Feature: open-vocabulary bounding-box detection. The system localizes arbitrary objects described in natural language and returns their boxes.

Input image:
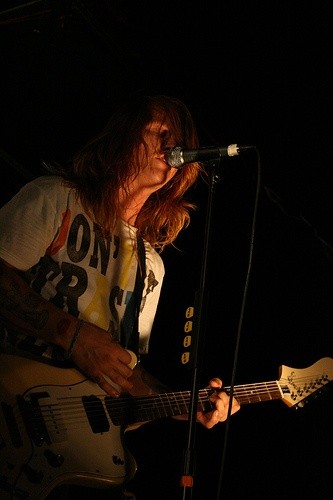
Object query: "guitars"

[1,355,333,496]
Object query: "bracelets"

[63,318,85,363]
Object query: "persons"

[0,93,242,500]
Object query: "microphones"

[164,143,258,169]
